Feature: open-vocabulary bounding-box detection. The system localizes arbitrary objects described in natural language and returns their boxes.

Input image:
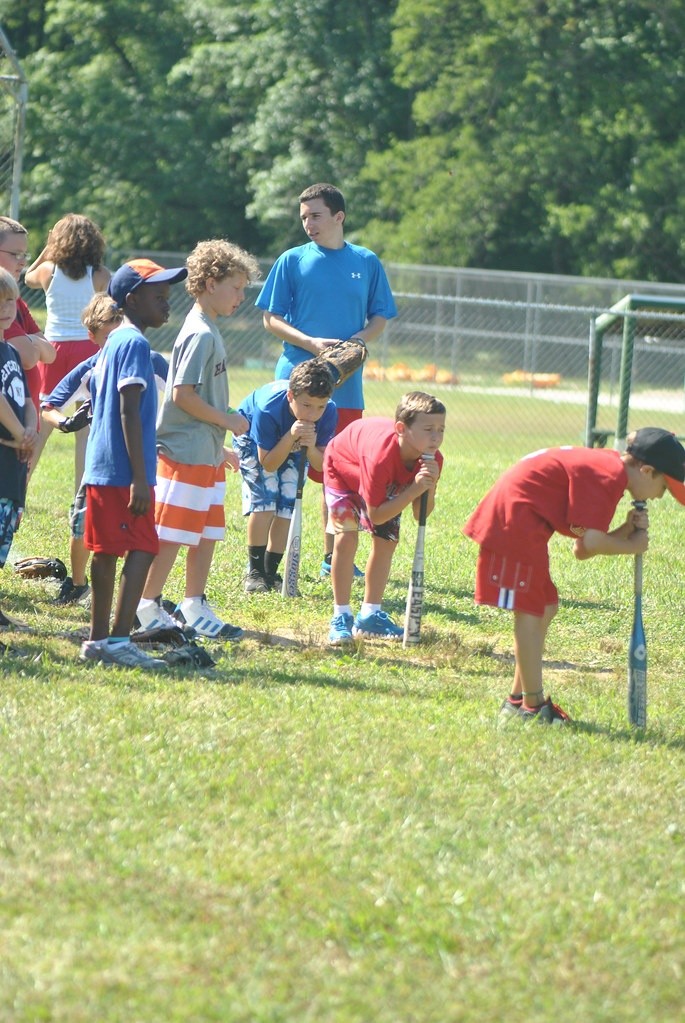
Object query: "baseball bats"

[628,501,647,726]
[403,455,437,644]
[281,446,308,599]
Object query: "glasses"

[0,249,32,262]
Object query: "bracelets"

[227,408,237,414]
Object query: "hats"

[628,426,685,509]
[108,259,189,307]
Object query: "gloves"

[59,398,92,433]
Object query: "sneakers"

[172,593,243,639]
[319,560,331,577]
[79,640,104,662]
[99,640,169,670]
[328,613,355,645]
[516,693,573,727]
[353,564,366,577]
[264,571,283,592]
[52,576,90,607]
[498,694,522,721]
[352,611,409,641]
[237,568,269,592]
[130,596,197,640]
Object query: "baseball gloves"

[319,338,368,387]
[156,641,217,670]
[13,556,67,580]
[131,625,198,648]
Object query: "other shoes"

[0,641,30,659]
[0,610,30,631]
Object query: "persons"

[0,265,38,655]
[39,292,240,610]
[463,428,685,725]
[0,216,57,533]
[322,391,446,646]
[79,258,171,669]
[131,239,263,641]
[231,359,338,591]
[254,183,398,577]
[24,213,111,497]
